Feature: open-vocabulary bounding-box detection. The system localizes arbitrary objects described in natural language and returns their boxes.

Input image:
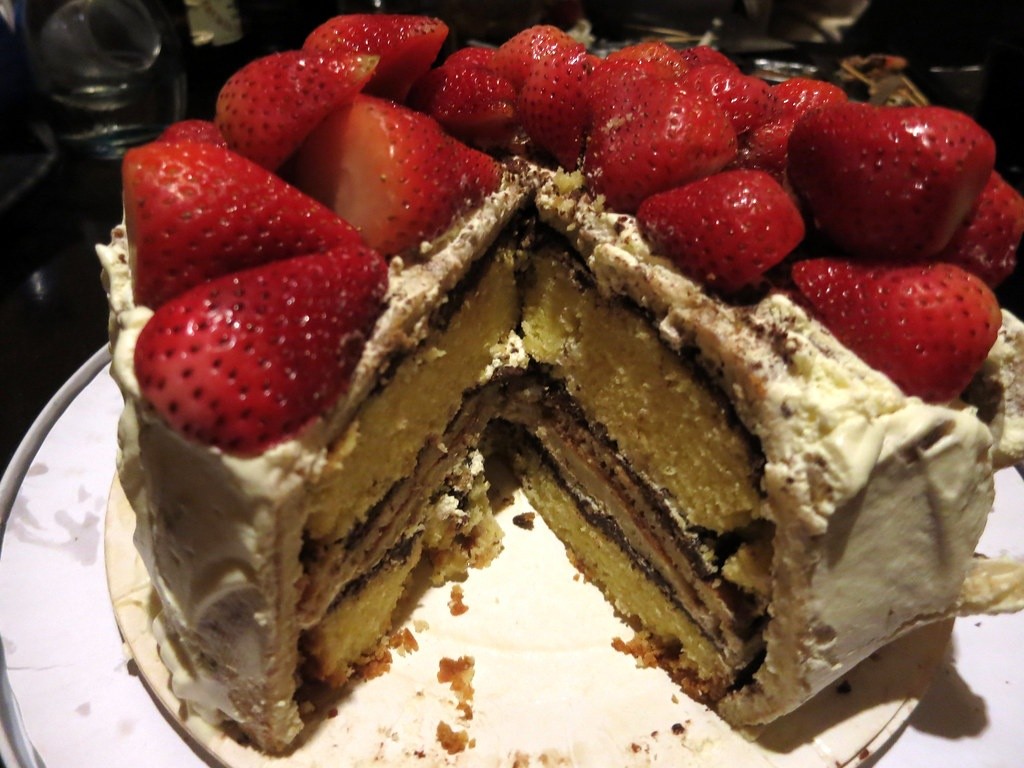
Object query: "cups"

[615,1,732,59]
[31,0,192,161]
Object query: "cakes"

[93,16,1024,752]
